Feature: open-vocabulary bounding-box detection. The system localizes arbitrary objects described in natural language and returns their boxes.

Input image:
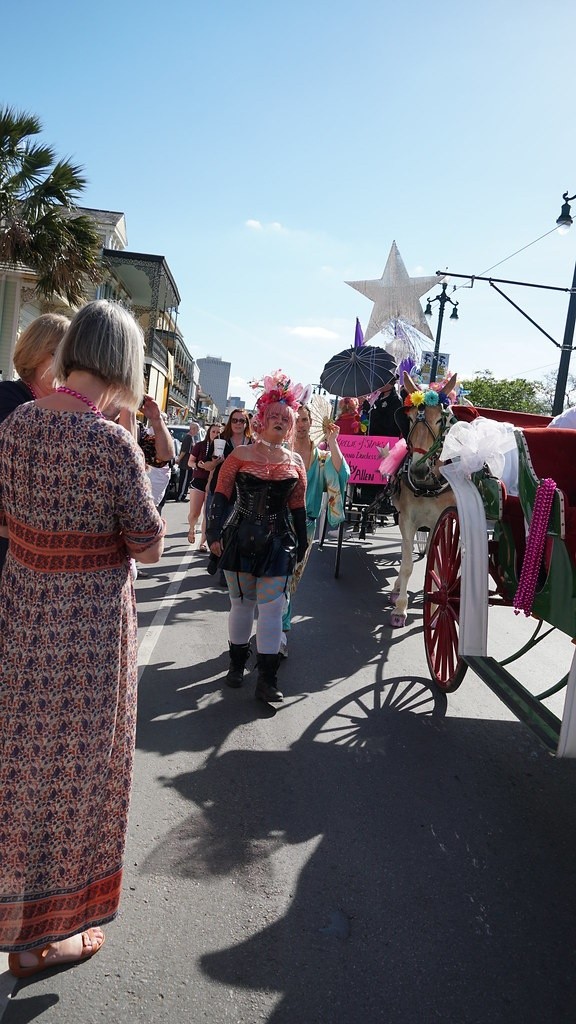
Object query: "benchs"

[448,405,554,521]
[512,428,576,540]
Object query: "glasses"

[232,418,247,424]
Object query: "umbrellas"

[320,346,396,397]
[355,323,363,347]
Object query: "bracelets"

[262,439,281,448]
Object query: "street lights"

[313,383,327,397]
[551,192,576,417]
[422,284,459,384]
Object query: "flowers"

[402,383,458,415]
[246,367,303,435]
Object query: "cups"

[215,438,226,459]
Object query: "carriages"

[316,370,475,577]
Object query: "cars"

[164,425,206,498]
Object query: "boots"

[255,652,285,702]
[226,641,253,688]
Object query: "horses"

[390,370,458,628]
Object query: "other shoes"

[176,498,189,502]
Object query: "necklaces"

[57,387,105,418]
[206,441,210,456]
[26,383,37,400]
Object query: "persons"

[334,374,404,436]
[172,394,350,702]
[0,301,177,976]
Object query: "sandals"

[188,530,195,543]
[200,544,207,552]
[9,928,105,978]
